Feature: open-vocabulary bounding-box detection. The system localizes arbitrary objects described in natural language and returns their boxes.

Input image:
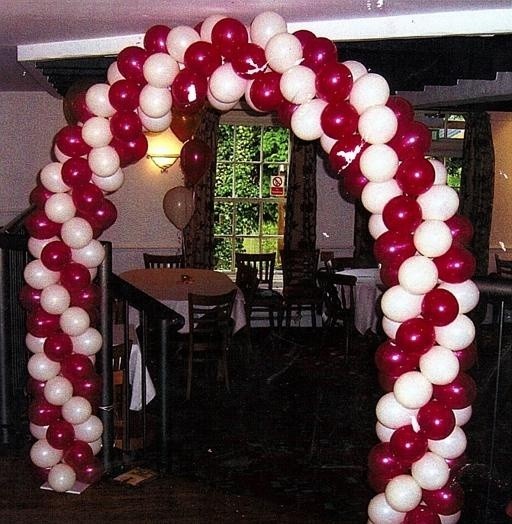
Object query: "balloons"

[366,256,481,524]
[138,9,369,140]
[27,395,104,492]
[32,47,149,233]
[19,217,104,406]
[318,73,474,284]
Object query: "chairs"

[232,249,373,365]
[490,254,512,338]
[186,288,239,399]
[140,252,190,268]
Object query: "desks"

[332,267,384,341]
[118,266,244,393]
